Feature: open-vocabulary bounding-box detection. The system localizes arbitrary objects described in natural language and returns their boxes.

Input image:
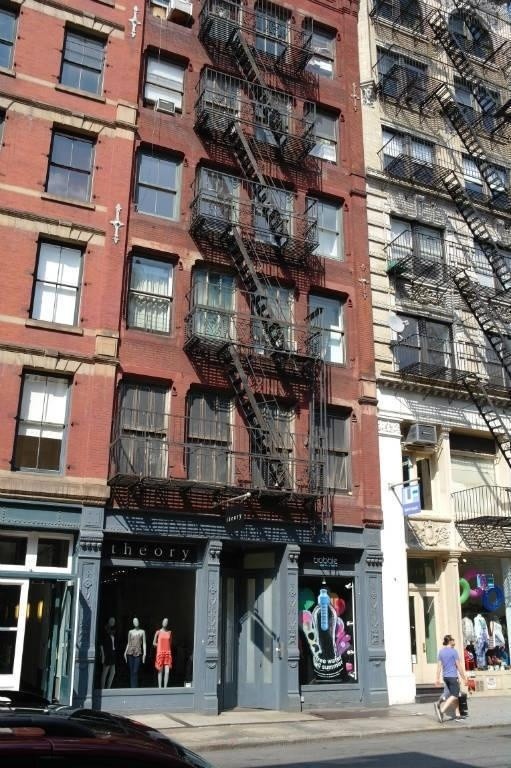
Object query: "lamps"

[313,559,327,584]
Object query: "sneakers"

[455,715,466,722]
[433,701,447,723]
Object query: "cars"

[1,689,219,768]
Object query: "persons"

[434,634,470,723]
[463,616,475,644]
[473,614,490,643]
[490,615,505,647]
[152,617,173,688]
[98,617,118,689]
[123,617,146,687]
